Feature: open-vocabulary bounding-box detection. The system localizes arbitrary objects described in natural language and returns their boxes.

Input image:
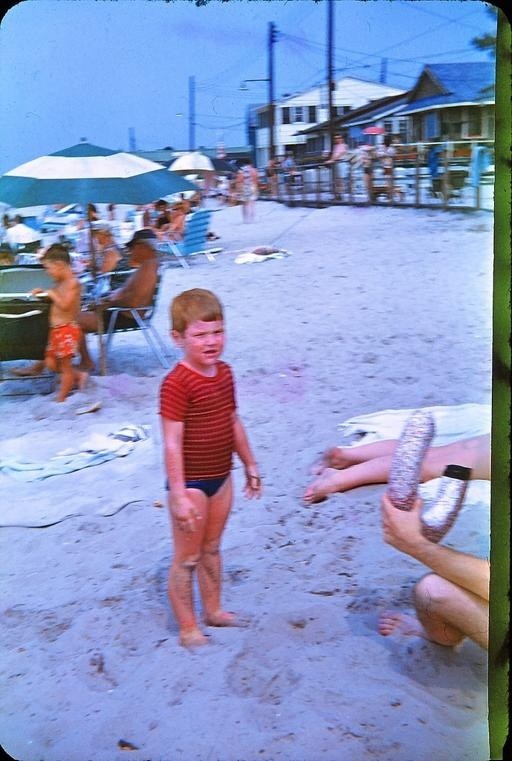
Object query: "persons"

[378,493,490,651]
[160,288,262,652]
[261,153,298,172]
[0,192,218,290]
[303,432,491,506]
[10,229,159,376]
[235,158,260,222]
[327,133,445,202]
[32,243,89,402]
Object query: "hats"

[126,229,157,247]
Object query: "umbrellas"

[0,136,203,276]
[169,146,239,177]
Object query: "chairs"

[160,206,218,267]
[104,259,174,368]
[0,291,54,395]
